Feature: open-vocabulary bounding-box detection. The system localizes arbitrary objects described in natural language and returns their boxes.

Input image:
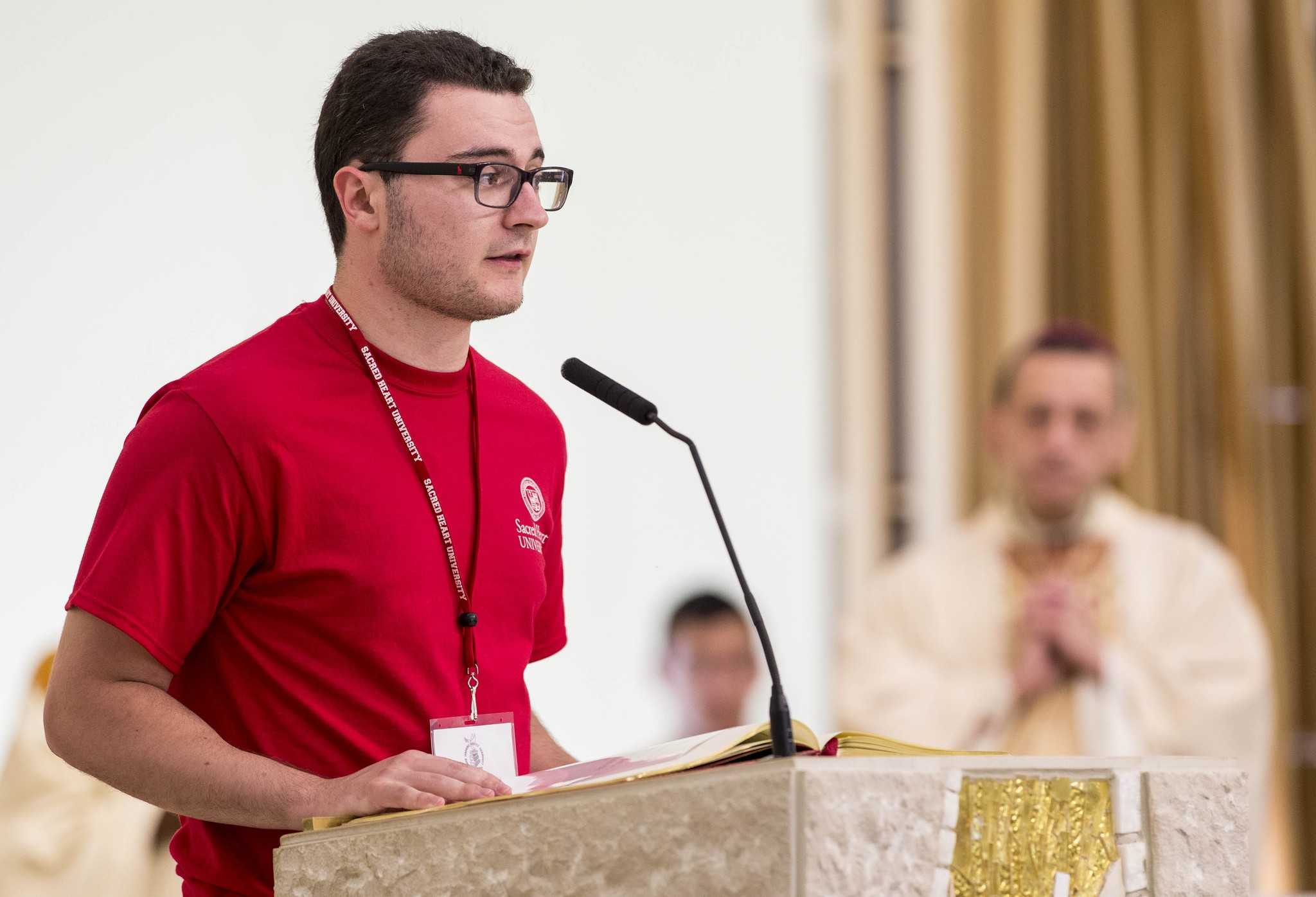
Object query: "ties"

[1006,536,1108,757]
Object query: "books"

[345,714,1012,824]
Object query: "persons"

[661,594,757,737]
[47,26,579,897]
[833,325,1279,768]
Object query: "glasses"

[354,161,574,212]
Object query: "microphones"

[559,356,795,761]
